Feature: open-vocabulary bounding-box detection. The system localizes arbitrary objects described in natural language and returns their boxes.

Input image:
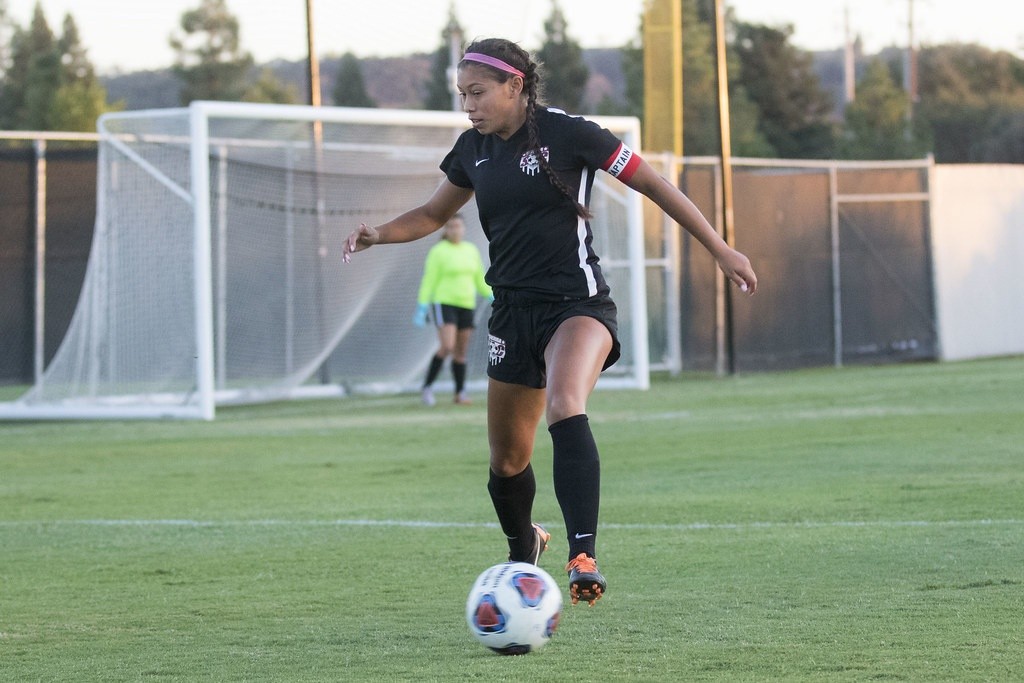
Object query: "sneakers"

[508,522,550,566]
[564,553,606,607]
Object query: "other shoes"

[423,385,437,405]
[455,395,472,405]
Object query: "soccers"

[462,560,565,657]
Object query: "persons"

[340,40,757,605]
[413,213,495,407]
